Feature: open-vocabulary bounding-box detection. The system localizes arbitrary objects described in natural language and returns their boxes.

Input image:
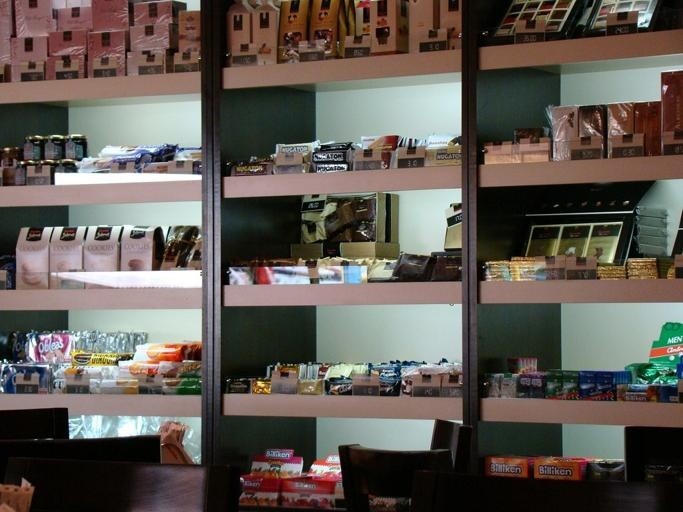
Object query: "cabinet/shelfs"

[0,0,202,466]
[476,0,682,464]
[221,0,463,471]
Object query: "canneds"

[3,134,88,186]
